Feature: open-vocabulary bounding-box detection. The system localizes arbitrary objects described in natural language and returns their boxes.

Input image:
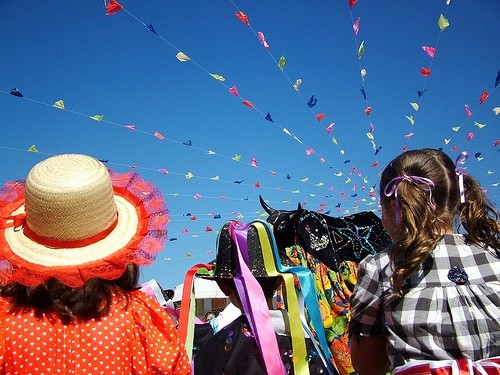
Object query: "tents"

[171,277,231,301]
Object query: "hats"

[0,153,168,287]
[195,221,302,278]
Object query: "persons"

[346,149,500,375]
[193,219,331,375]
[0,152,194,374]
[162,288,284,338]
